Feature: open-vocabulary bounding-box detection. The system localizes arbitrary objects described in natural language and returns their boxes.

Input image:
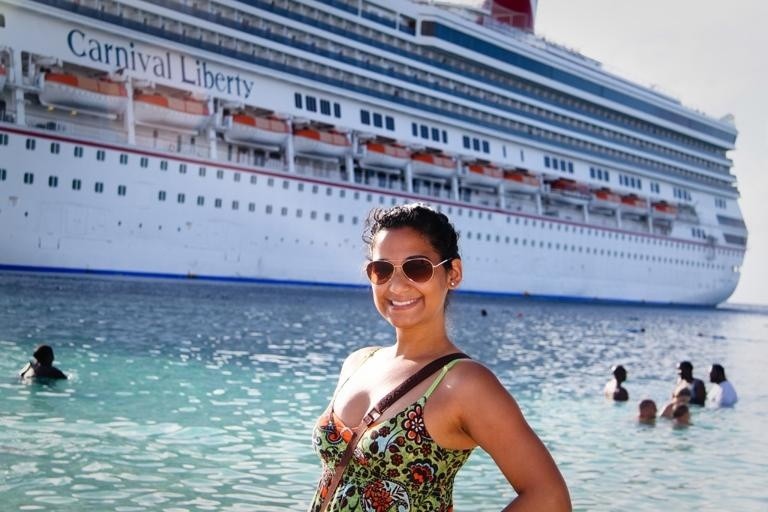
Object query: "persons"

[604,362,737,430]
[308,202,572,512]
[18,345,67,383]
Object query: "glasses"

[364,257,452,286]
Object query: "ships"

[1,0,750,311]
